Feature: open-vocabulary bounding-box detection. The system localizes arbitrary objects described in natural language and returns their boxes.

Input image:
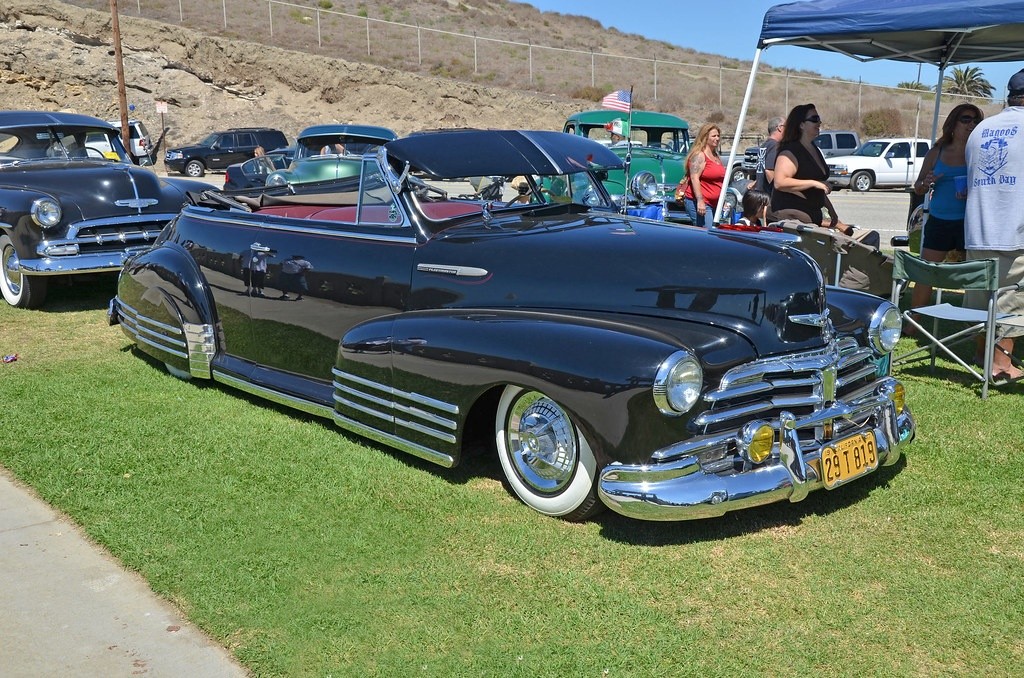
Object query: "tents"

[713,0,1024,228]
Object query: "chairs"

[420,202,484,219]
[767,221,893,304]
[304,205,400,224]
[884,244,1024,397]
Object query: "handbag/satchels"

[676,179,689,207]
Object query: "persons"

[320,144,350,155]
[734,189,770,226]
[964,68,1024,379]
[254,146,276,174]
[683,124,726,228]
[277,254,314,301]
[470,175,503,201]
[606,117,628,137]
[602,89,631,113]
[611,132,630,145]
[238,244,277,297]
[900,104,985,336]
[747,103,854,236]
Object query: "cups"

[954,176,967,195]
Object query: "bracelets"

[921,180,925,188]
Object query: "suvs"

[163,127,290,177]
[84,120,153,158]
[744,131,861,192]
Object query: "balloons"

[129,104,135,111]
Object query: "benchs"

[250,205,337,220]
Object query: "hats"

[604,118,627,136]
[511,176,529,190]
[1008,69,1024,90]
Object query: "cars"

[529,108,692,224]
[0,110,223,310]
[223,124,398,191]
[824,138,932,193]
[107,128,916,524]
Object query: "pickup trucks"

[667,139,748,187]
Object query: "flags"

[610,228,636,236]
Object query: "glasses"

[804,115,820,123]
[957,115,982,124]
